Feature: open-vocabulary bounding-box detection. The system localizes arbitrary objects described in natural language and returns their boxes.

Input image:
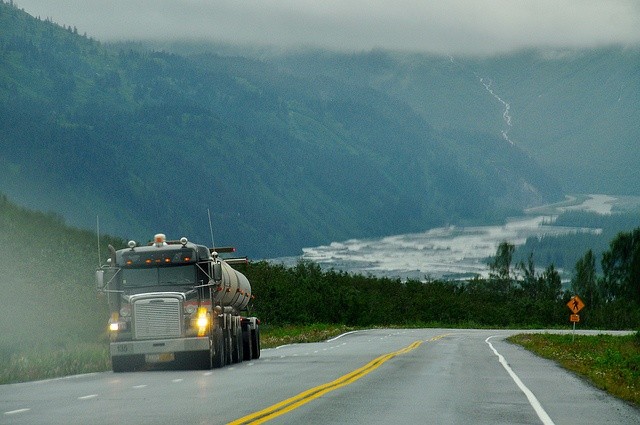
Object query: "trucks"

[95,207,260,372]
